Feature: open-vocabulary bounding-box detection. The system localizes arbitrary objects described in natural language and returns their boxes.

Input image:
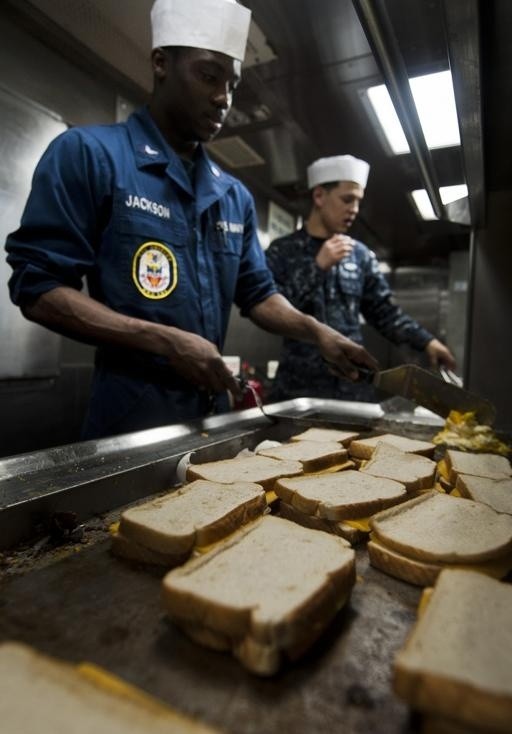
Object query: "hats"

[150,0,252,63]
[307,154,371,190]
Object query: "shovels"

[234,378,369,433]
[279,342,496,427]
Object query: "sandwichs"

[111,426,512,734]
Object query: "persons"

[259,151,461,409]
[2,0,381,447]
